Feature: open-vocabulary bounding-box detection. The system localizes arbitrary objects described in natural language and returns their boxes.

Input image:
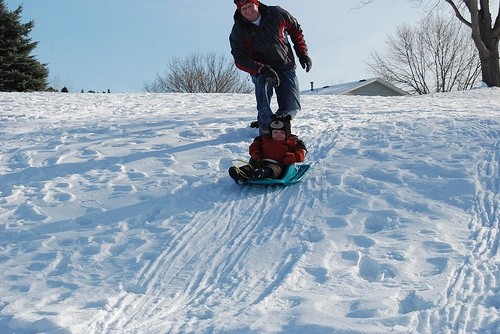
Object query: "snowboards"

[246,164,312,187]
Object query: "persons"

[229,0,313,134]
[229,115,308,181]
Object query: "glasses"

[241,4,256,10]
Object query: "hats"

[234,0,259,11]
[268,114,292,140]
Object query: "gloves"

[260,65,280,87]
[282,152,296,165]
[299,55,312,72]
[252,152,260,160]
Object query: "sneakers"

[228,166,246,185]
[252,168,265,180]
